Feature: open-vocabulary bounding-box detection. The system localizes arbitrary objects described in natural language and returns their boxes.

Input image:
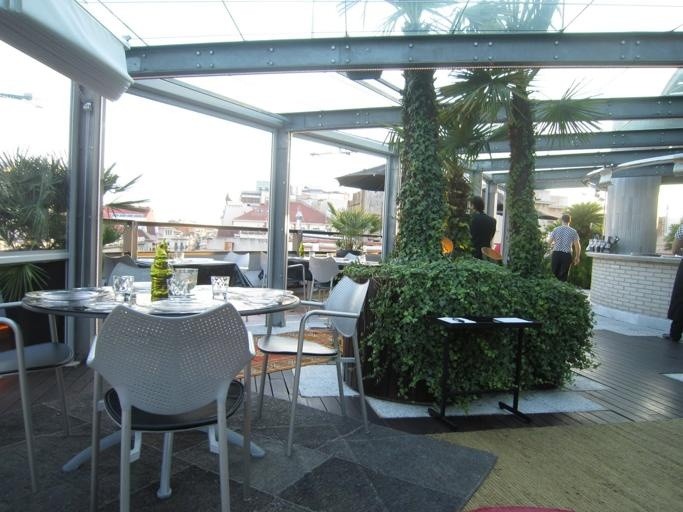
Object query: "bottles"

[588,235,612,253]
[299,241,304,257]
[150,240,172,301]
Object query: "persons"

[467,196,497,261]
[659,218,683,342]
[546,214,581,283]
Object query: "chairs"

[480,245,503,267]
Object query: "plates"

[25,288,108,304]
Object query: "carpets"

[0,384,497,512]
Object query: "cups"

[210,275,231,302]
[110,274,135,303]
[174,266,198,298]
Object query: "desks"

[426,313,542,426]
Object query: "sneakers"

[663,334,678,344]
